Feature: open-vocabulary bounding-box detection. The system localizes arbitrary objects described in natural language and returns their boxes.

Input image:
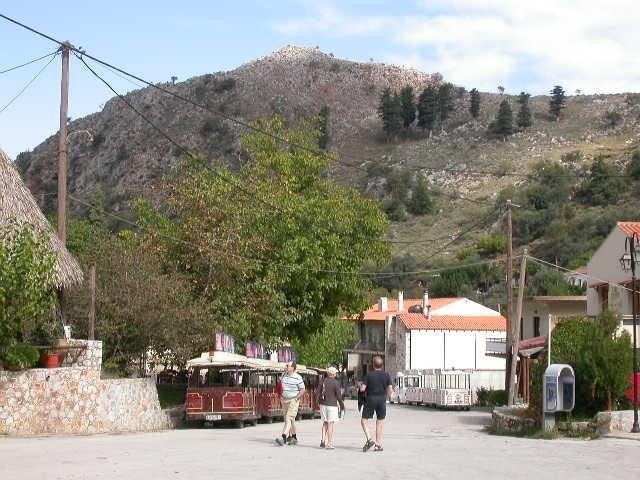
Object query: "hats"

[327,366,339,374]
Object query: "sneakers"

[276,435,298,447]
[320,441,335,449]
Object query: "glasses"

[286,364,295,368]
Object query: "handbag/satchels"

[276,383,283,400]
[314,393,326,401]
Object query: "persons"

[318,367,344,449]
[358,356,393,452]
[276,361,305,446]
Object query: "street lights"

[619,230,640,434]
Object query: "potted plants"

[37,290,70,367]
[4,345,39,371]
[33,313,60,368]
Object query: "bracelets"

[295,398,298,402]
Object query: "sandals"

[362,439,376,452]
[375,443,384,451]
